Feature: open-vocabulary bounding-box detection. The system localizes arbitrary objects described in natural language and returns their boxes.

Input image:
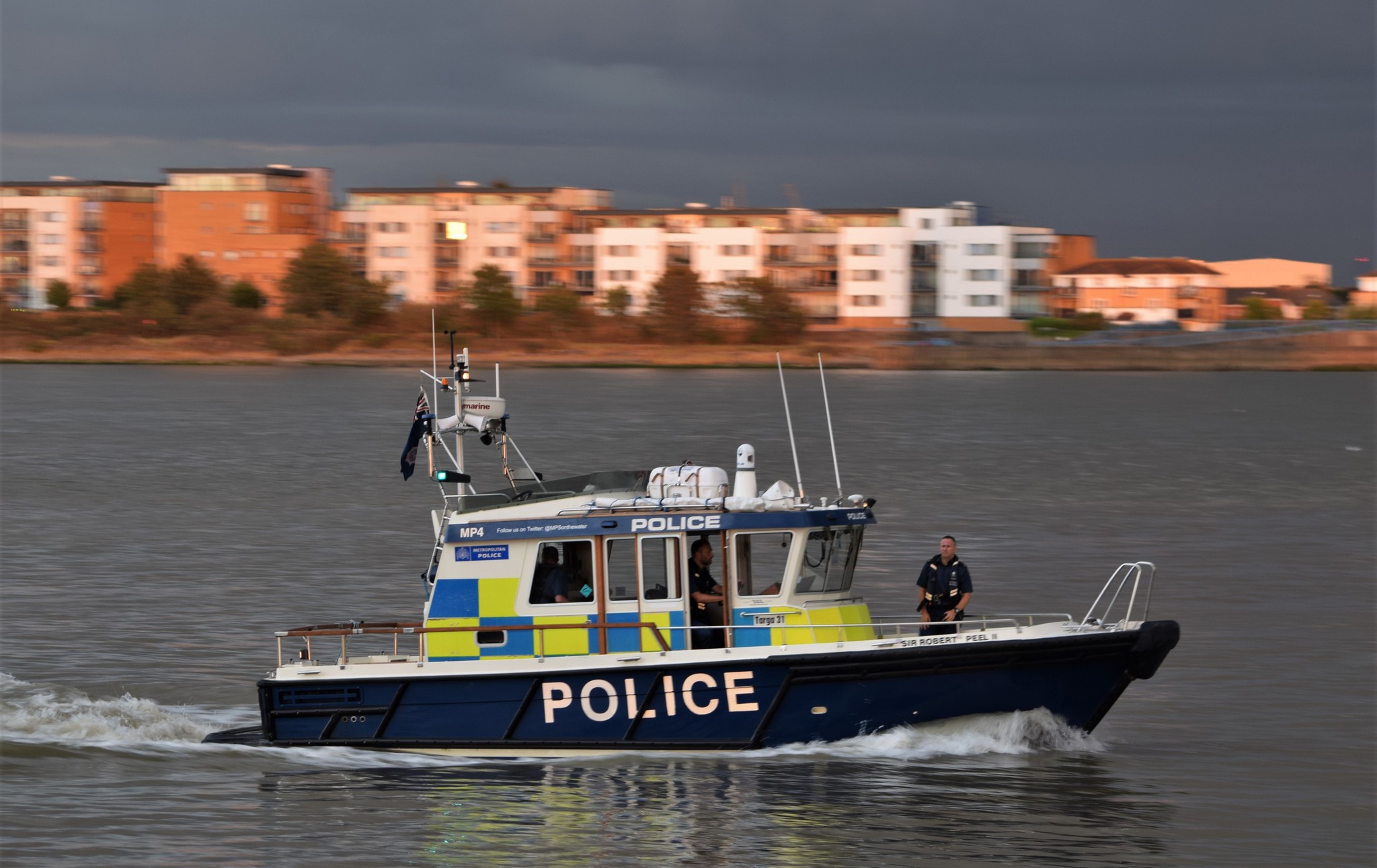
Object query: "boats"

[200,309,1182,758]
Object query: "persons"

[680,540,744,649]
[530,545,571,604]
[917,535,973,636]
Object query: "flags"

[400,390,432,482]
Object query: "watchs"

[953,607,959,614]
